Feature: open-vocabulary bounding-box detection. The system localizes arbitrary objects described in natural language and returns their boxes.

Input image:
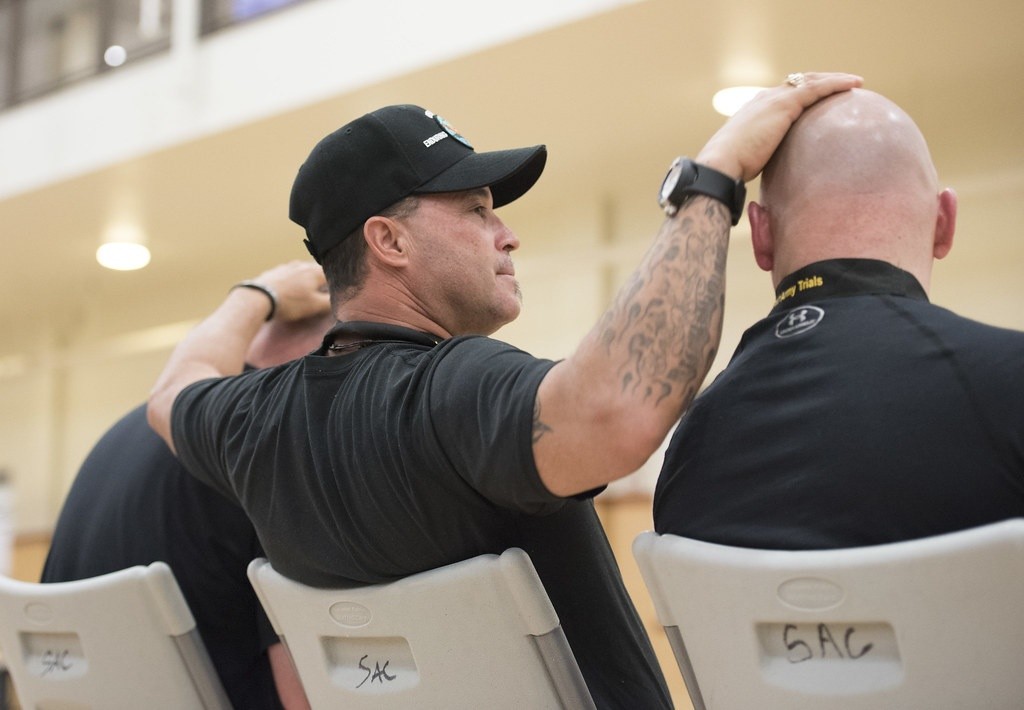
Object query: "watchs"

[660,157,747,226]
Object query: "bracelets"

[230,281,277,322]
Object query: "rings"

[787,74,803,81]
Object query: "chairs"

[631,519,1024,710]
[0,561,232,710]
[248,551,597,710]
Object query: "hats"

[287,103,549,265]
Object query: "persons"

[652,86,1024,554]
[144,70,864,709]
[32,283,313,708]
